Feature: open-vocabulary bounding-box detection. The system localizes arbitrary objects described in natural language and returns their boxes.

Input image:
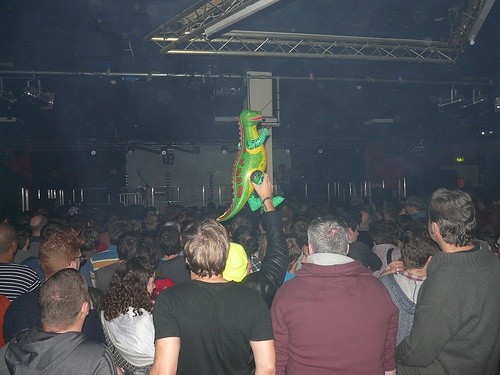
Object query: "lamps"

[0,78,18,105]
[20,74,56,110]
[161,146,167,155]
[438,83,489,113]
[284,144,291,155]
[317,144,325,155]
[221,144,228,155]
[90,144,97,156]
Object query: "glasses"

[69,254,86,267]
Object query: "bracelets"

[263,197,273,203]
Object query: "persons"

[146,219,274,375]
[0,187,500,375]
